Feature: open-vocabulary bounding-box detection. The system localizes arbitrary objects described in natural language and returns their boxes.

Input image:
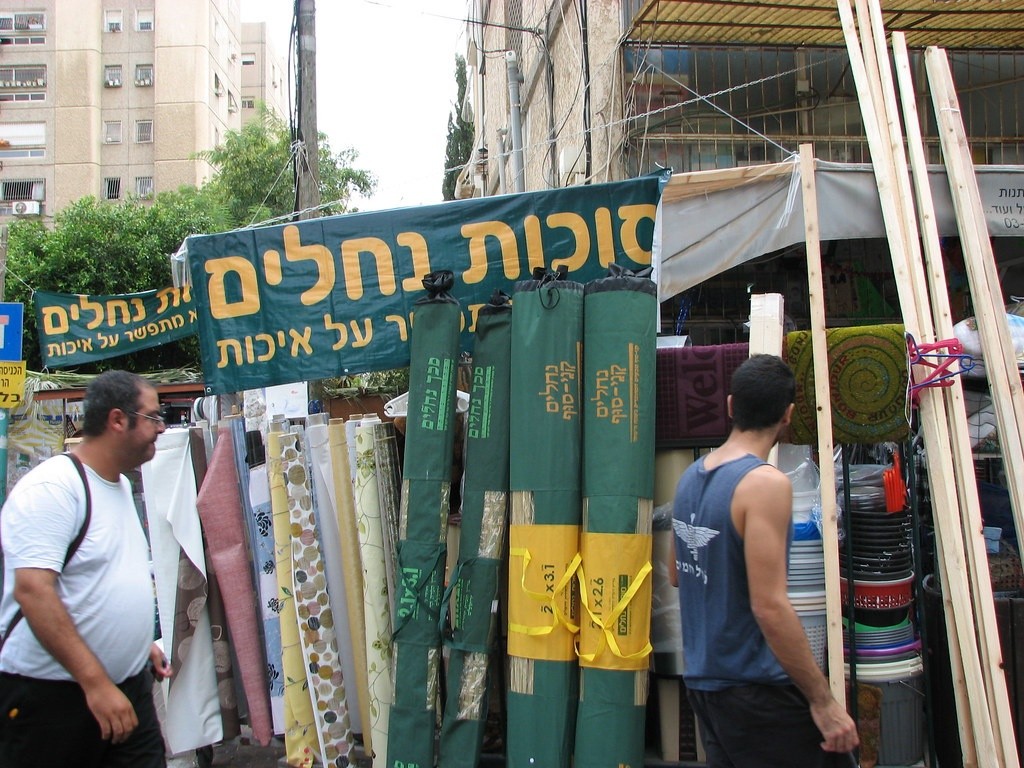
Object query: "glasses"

[118,407,167,430]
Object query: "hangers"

[905,332,975,409]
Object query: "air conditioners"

[12,201,40,216]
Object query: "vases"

[109,79,120,86]
[0,77,43,87]
[135,78,151,85]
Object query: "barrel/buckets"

[838,503,925,766]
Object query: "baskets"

[784,463,927,682]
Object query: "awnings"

[617,0,1024,155]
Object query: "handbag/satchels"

[987,540,1023,591]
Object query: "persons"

[669,356,861,768]
[0,368,174,768]
